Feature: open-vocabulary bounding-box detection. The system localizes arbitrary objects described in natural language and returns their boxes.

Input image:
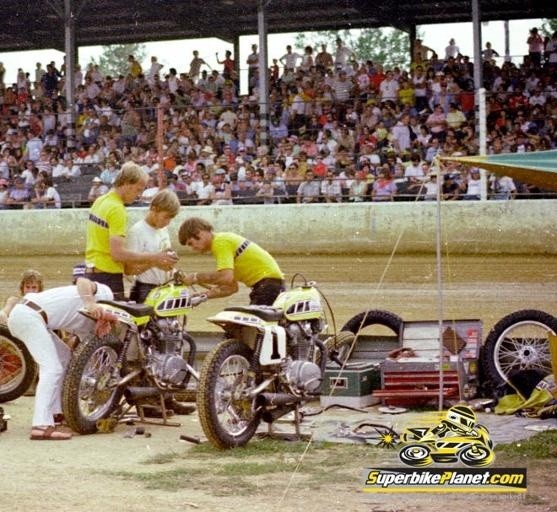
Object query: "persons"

[6,276,126,438]
[0,27,557,210]
[0,271,43,327]
[124,192,197,418]
[173,218,287,312]
[81,158,179,300]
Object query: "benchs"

[178,181,428,205]
[52,162,101,207]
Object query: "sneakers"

[137,405,173,418]
[165,400,195,414]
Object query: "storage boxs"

[372,318,483,408]
[323,360,375,396]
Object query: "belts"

[16,298,48,324]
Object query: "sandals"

[31,426,72,440]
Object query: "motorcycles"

[60,266,198,436]
[195,282,359,452]
[397,406,494,468]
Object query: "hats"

[242,156,251,163]
[201,145,213,154]
